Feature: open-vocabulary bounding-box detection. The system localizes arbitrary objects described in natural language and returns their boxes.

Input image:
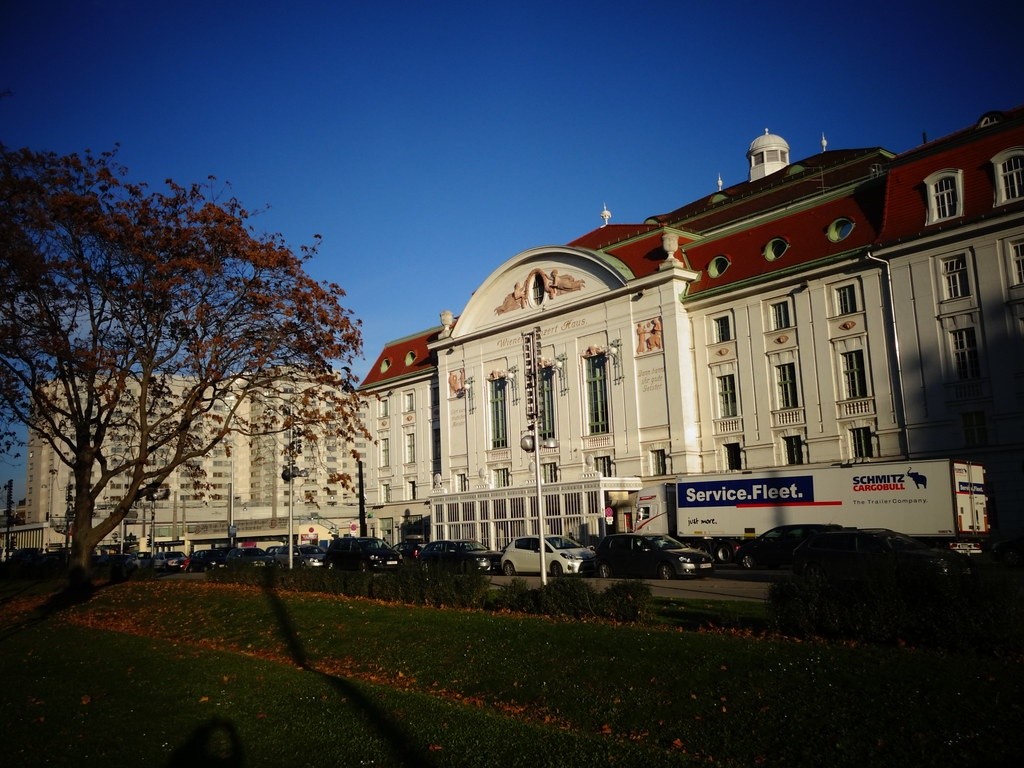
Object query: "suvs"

[322,535,404,573]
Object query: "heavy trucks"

[631,458,991,571]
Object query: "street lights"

[520,335,559,587]
[282,403,309,570]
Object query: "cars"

[594,532,716,580]
[734,523,844,572]
[90,543,325,573]
[391,540,425,564]
[791,526,974,576]
[991,535,1024,569]
[415,540,503,575]
[500,532,596,578]
